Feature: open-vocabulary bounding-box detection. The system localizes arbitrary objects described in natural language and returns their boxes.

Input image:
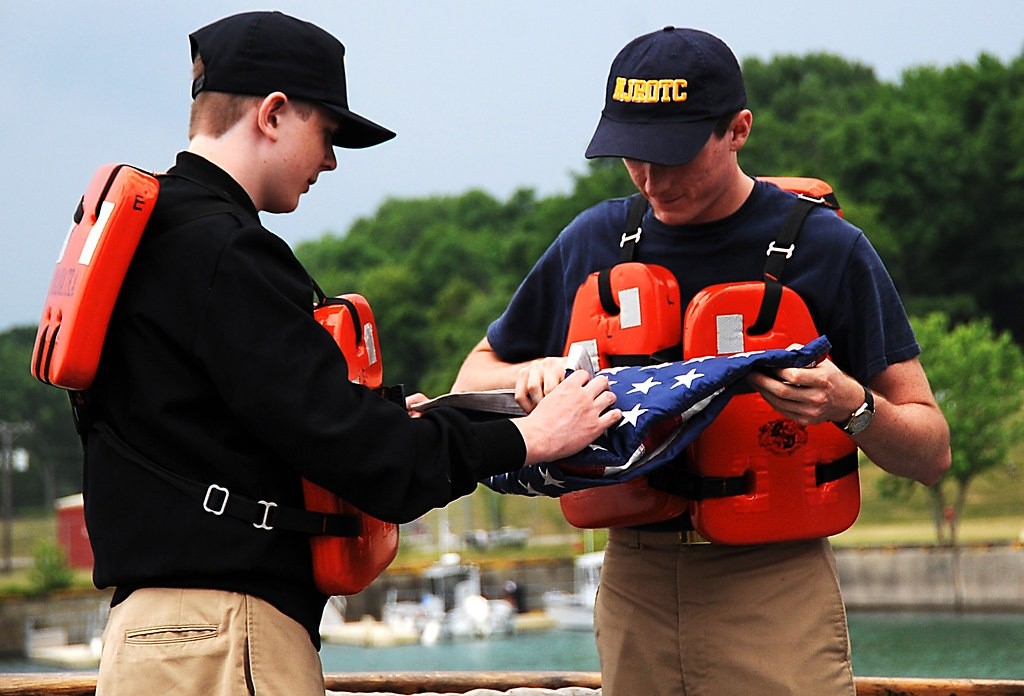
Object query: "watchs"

[834,388,876,436]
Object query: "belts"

[636,517,715,545]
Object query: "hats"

[188,12,396,149]
[585,26,744,168]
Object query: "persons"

[67,10,620,696]
[452,26,952,696]
